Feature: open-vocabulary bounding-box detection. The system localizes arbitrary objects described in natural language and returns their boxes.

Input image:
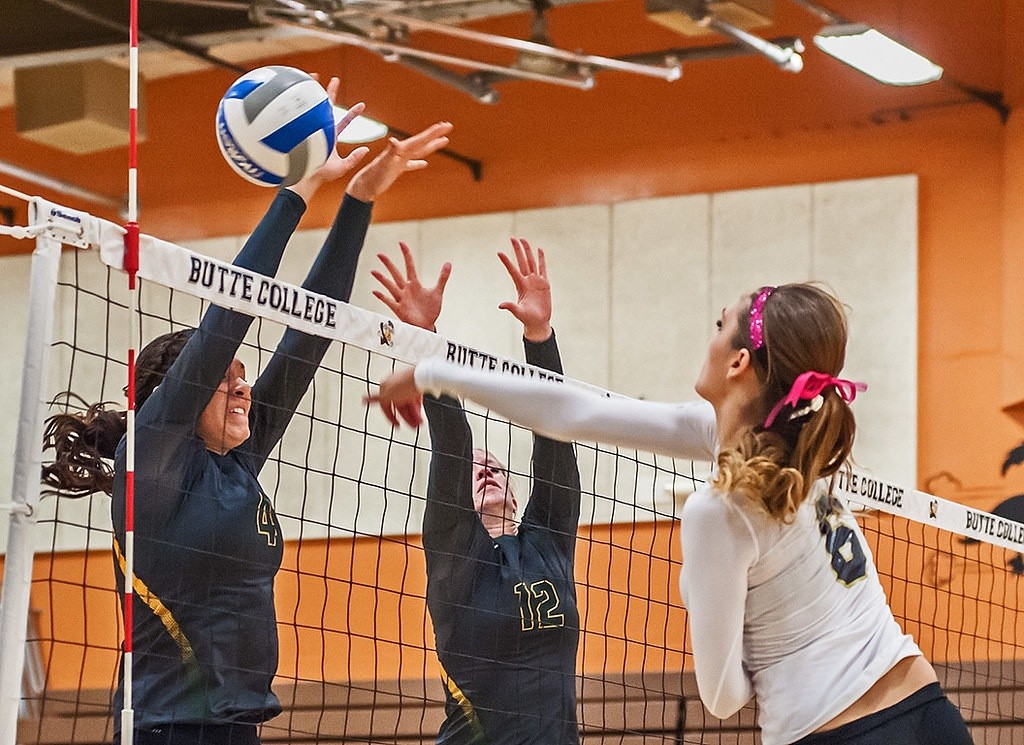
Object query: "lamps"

[813,21,944,87]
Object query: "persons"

[114,74,455,745]
[361,283,973,745]
[371,236,582,745]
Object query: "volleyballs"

[215,65,336,189]
